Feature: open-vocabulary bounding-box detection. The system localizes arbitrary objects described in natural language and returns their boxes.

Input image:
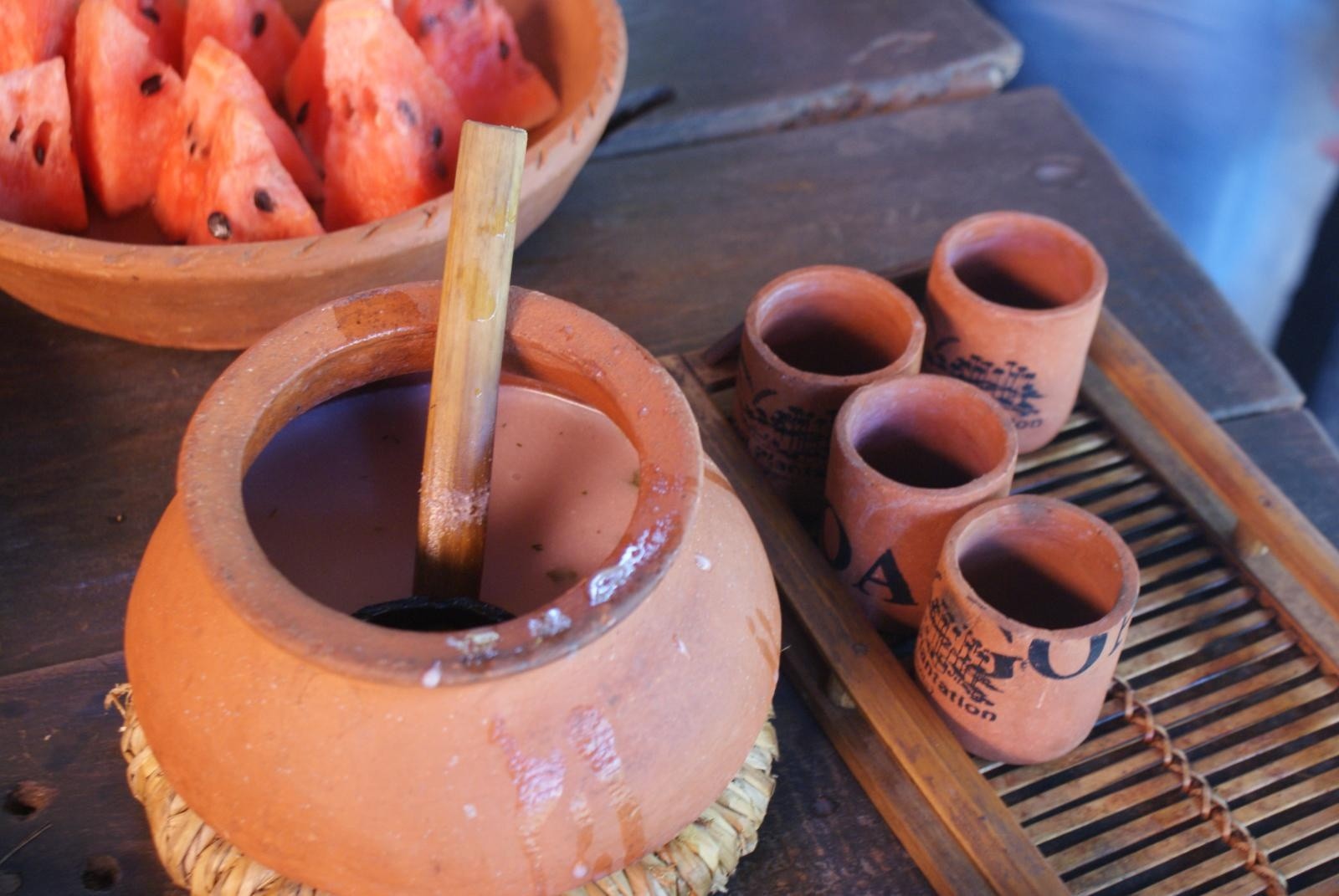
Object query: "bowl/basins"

[1,0,627,352]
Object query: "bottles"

[121,276,782,896]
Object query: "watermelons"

[0,0,556,243]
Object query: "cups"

[743,209,1141,767]
